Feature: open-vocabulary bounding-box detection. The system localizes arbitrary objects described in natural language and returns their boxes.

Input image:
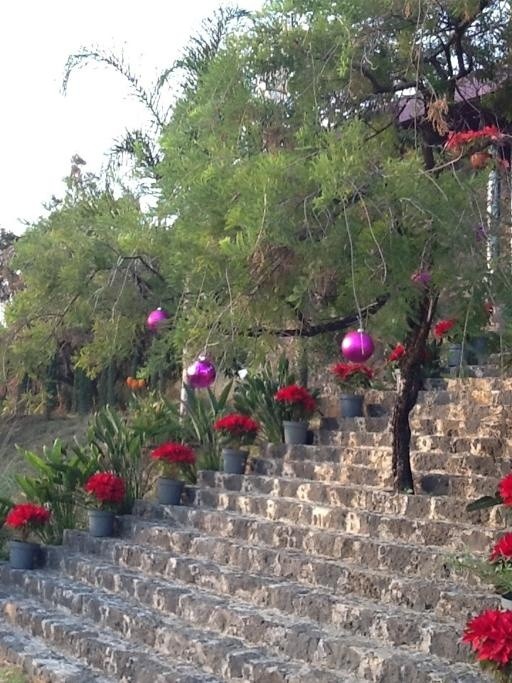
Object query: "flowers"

[333,363,373,393]
[151,443,195,479]
[213,415,258,449]
[433,318,469,346]
[5,504,49,541]
[275,386,316,421]
[86,473,124,509]
[459,474,512,683]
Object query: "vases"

[283,421,309,443]
[447,348,471,367]
[88,511,115,537]
[223,449,249,474]
[8,542,39,569]
[157,479,184,505]
[339,395,364,417]
